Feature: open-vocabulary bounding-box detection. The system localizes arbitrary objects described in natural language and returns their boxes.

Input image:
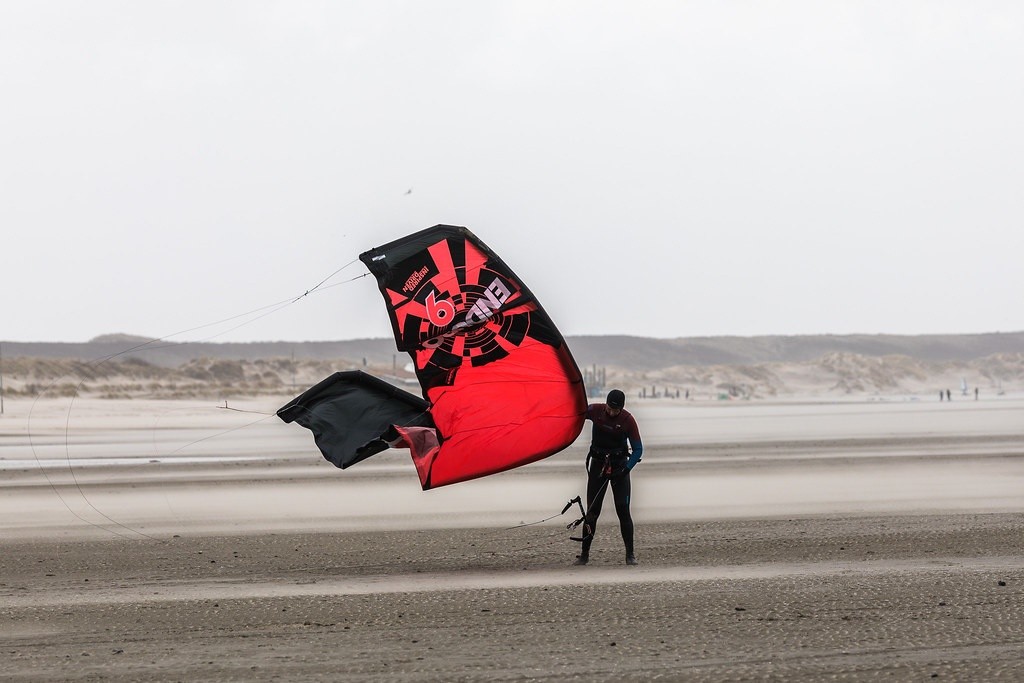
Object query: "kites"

[277,224,588,491]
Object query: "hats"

[607,390,625,409]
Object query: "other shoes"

[573,554,588,566]
[626,554,638,565]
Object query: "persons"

[937,386,979,401]
[571,389,643,566]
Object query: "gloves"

[606,466,629,481]
